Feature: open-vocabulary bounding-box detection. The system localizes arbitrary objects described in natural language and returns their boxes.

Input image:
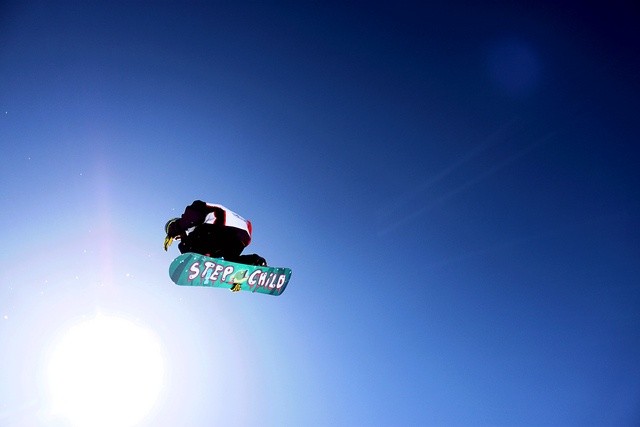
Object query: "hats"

[166,218,180,234]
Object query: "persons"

[163,199,268,268]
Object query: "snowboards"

[168,252,292,296]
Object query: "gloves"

[164,236,173,251]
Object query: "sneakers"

[204,251,224,260]
[252,255,267,267]
